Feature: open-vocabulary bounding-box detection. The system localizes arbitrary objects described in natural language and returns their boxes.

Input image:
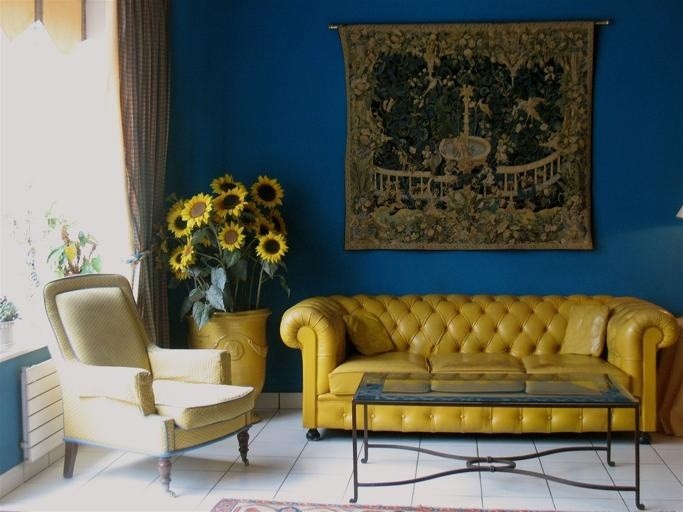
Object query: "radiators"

[20,358,65,464]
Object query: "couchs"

[277,292,681,445]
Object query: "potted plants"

[0,295,24,351]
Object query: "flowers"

[128,172,293,348]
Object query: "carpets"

[208,497,558,512]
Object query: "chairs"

[37,272,257,492]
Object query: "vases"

[182,306,274,425]
